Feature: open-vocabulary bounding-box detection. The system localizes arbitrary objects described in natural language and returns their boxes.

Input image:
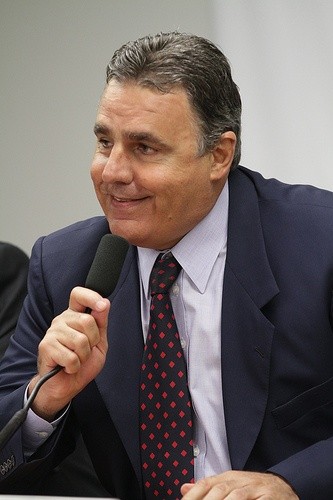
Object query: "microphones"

[82,235,130,314]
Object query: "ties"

[139,256,194,500]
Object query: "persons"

[0,32,333,500]
